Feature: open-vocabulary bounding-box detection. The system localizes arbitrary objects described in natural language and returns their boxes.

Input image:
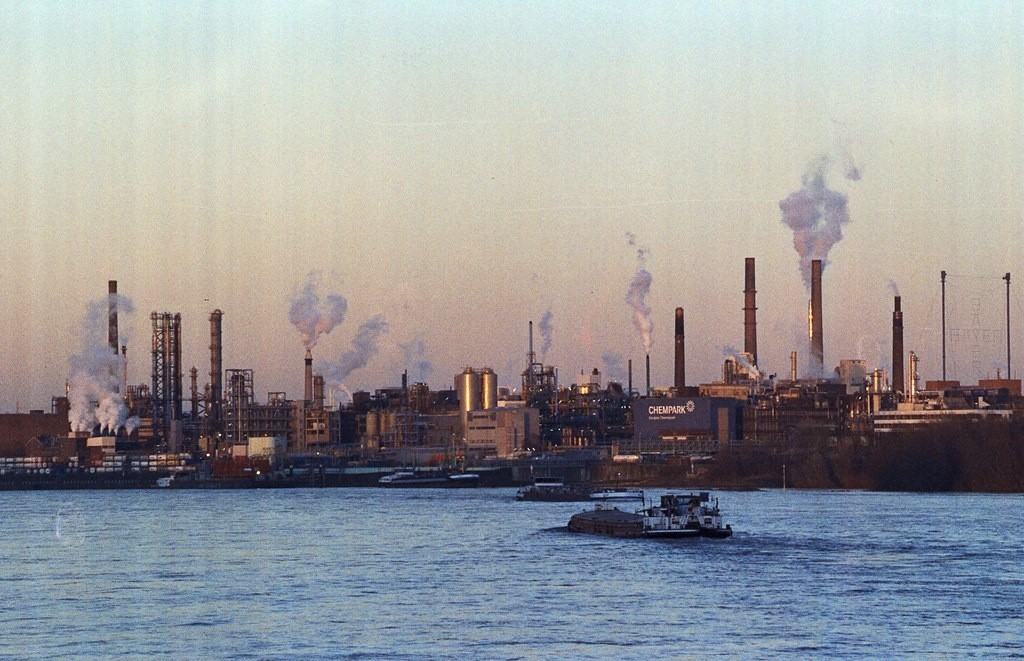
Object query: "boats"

[376,448,479,487]
[640,488,735,538]
[589,473,645,503]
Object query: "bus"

[919,387,1010,409]
